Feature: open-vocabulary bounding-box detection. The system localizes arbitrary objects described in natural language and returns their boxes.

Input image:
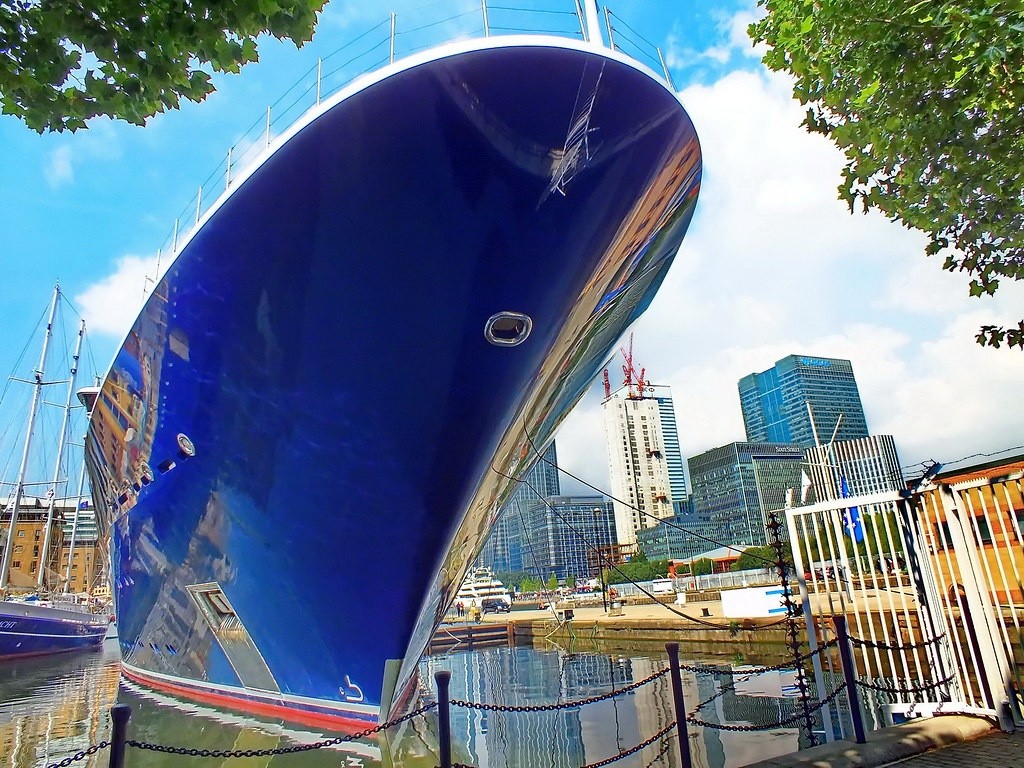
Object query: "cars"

[802,564,843,581]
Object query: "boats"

[74,0,707,732]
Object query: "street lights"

[593,507,608,613]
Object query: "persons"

[517,590,557,610]
[456,602,464,617]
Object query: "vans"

[481,598,511,614]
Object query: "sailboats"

[1,277,118,660]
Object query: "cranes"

[621,332,634,387]
[620,347,646,401]
[602,368,611,398]
